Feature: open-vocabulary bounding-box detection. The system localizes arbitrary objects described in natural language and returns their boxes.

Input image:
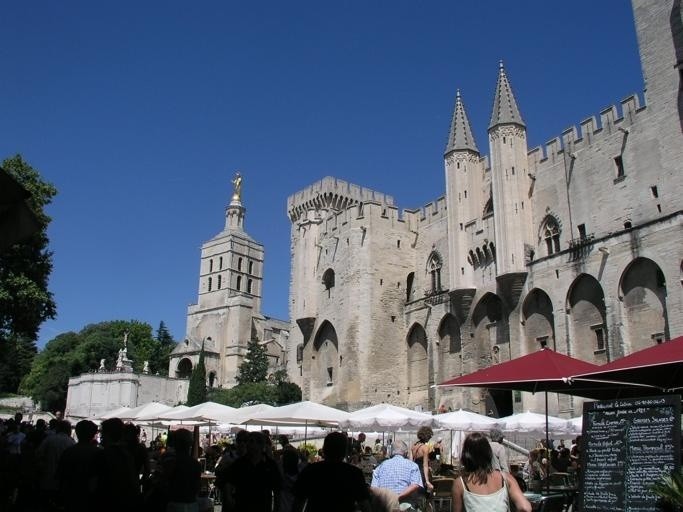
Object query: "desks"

[200,474,217,499]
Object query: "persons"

[1,413,423,512]
[411,427,584,512]
[229,170,242,200]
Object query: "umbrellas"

[437,349,662,499]
[566,335,682,390]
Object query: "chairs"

[536,496,573,512]
[432,478,455,512]
